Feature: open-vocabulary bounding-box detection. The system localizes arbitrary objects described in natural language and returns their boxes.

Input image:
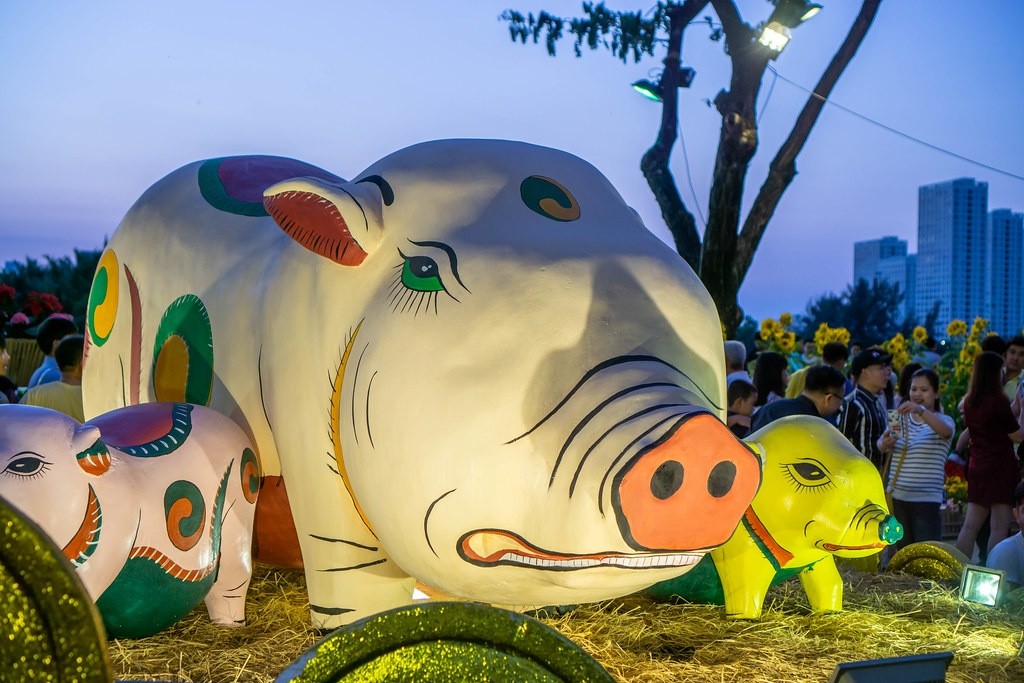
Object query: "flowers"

[720,311,1001,509]
[0,283,66,337]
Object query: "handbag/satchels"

[881,489,894,516]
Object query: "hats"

[851,349,894,371]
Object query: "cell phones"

[888,409,904,438]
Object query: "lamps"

[829,650,956,683]
[796,3,823,23]
[958,563,1012,611]
[631,76,664,103]
[753,19,792,62]
[675,67,698,89]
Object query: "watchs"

[918,404,926,416]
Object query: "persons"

[835,349,894,473]
[743,364,849,438]
[18,334,85,425]
[751,330,945,415]
[876,367,956,552]
[955,352,1024,561]
[724,340,753,389]
[727,414,751,429]
[986,480,1024,594]
[26,317,79,391]
[0,334,17,404]
[956,335,1024,477]
[727,379,759,439]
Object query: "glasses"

[825,392,847,407]
